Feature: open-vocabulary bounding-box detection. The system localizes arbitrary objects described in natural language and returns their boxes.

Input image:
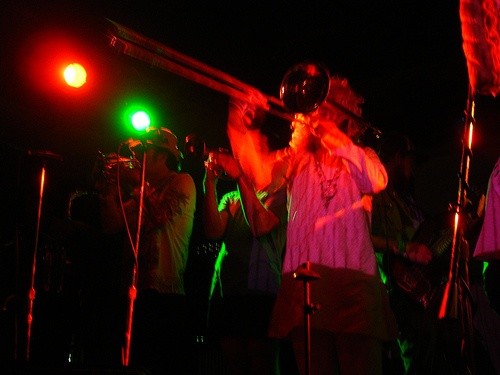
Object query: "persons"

[84,73,500,375]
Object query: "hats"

[145,128,184,159]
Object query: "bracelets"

[385,237,390,253]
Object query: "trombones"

[110,25,382,149]
